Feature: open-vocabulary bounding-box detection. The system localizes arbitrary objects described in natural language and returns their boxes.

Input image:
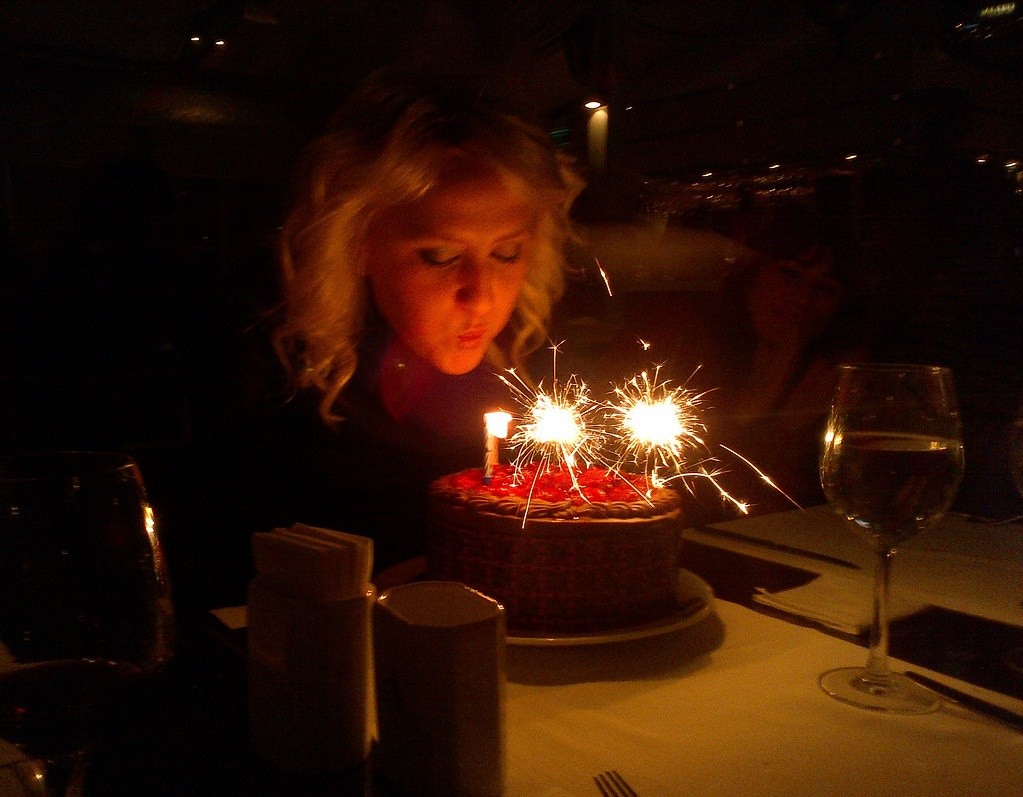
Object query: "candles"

[491,413,512,465]
[482,413,494,485]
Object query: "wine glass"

[817,362,966,715]
[1,451,175,797]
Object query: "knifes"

[904,671,1023,735]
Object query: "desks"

[203,505,1023,797]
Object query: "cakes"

[424,460,682,633]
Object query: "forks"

[591,768,638,797]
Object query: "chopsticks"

[692,523,861,572]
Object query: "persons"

[122,79,582,649]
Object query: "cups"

[247,577,379,775]
[372,580,505,797]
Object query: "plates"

[378,556,714,648]
